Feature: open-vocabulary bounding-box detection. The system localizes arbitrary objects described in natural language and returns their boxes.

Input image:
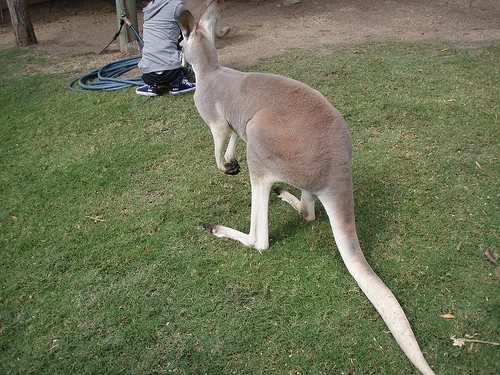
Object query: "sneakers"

[168,78,196,94]
[135,84,159,96]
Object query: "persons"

[135,0,195,96]
